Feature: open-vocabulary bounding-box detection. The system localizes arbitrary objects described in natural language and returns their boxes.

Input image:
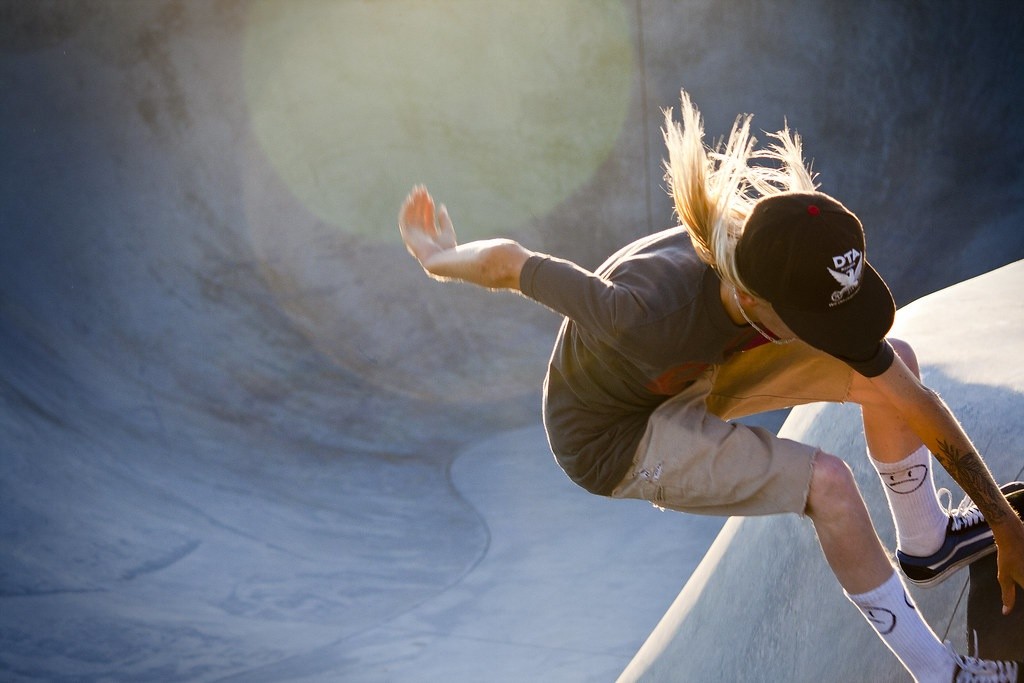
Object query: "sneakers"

[944,628,1024,683]
[895,482,1023,588]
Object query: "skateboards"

[965,479,1024,662]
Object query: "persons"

[398,88,1024,683]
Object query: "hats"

[742,192,897,357]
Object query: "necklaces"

[733,286,797,345]
